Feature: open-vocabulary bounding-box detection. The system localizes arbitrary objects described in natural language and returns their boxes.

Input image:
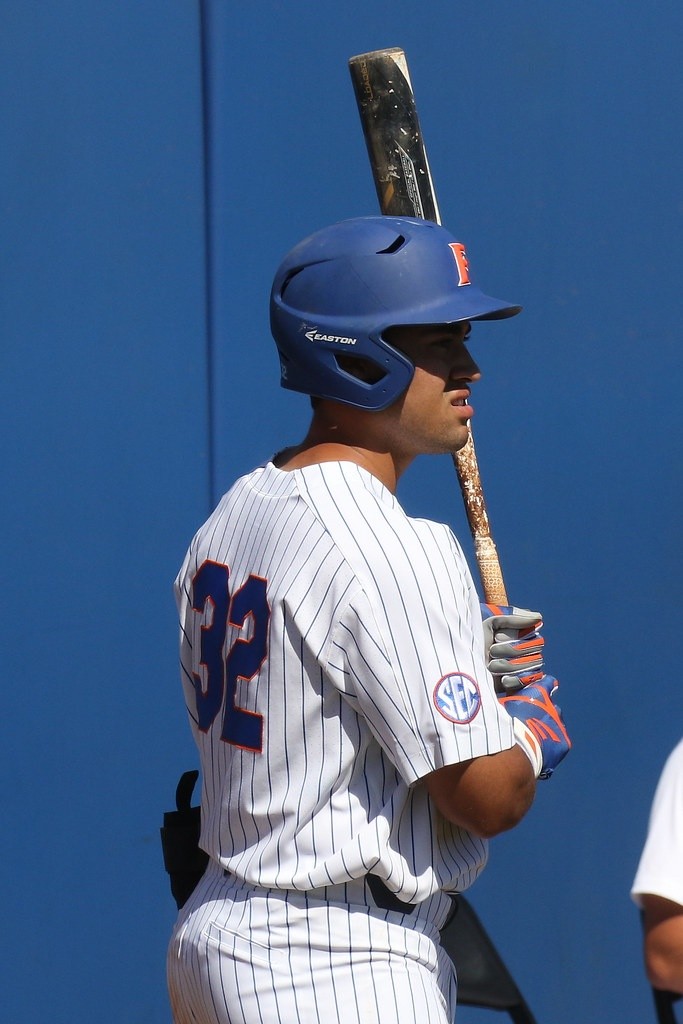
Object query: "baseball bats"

[350,45,506,613]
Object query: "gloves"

[497,674,573,781]
[479,603,545,694]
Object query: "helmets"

[269,215,525,411]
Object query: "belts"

[223,870,459,932]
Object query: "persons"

[626,739,683,1023]
[168,211,572,1023]
